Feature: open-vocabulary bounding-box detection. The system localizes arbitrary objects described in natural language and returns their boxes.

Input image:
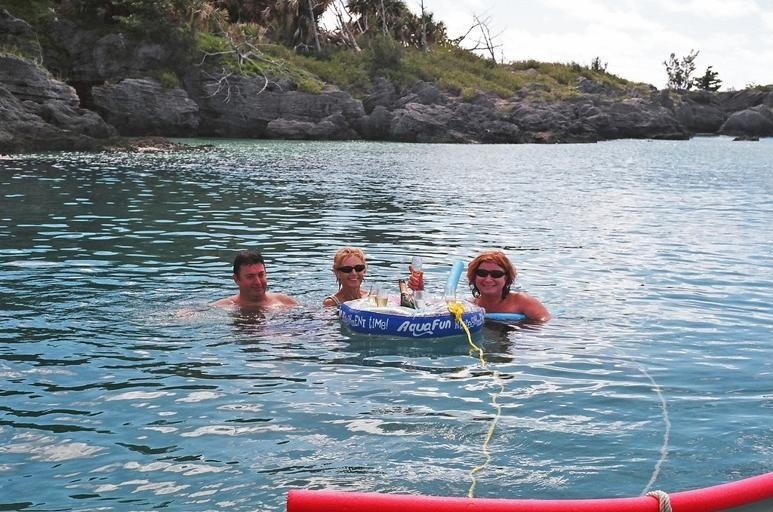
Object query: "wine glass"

[412,256,423,297]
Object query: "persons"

[323,247,372,307]
[208,252,297,309]
[408,252,549,322]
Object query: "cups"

[366,283,389,309]
[425,286,458,313]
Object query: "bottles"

[398,279,415,309]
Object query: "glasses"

[475,268,507,279]
[336,263,367,273]
